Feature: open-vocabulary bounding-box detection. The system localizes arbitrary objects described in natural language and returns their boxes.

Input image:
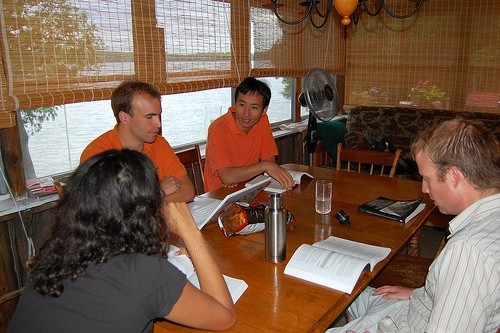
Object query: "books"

[245,169,314,194]
[167,244,248,304]
[284,236,391,294]
[357,195,425,223]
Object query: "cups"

[315,179,332,214]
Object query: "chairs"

[175,144,205,204]
[337,142,401,178]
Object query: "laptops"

[187,177,271,231]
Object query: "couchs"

[346,107,500,228]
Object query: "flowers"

[407,80,449,109]
[351,85,390,106]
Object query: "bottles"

[265,193,287,265]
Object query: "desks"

[154,164,439,333]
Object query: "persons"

[204,77,296,193]
[325,117,500,333]
[80,81,195,205]
[7,149,238,333]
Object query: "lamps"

[271,0,424,28]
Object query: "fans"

[298,68,340,167]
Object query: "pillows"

[317,119,347,162]
